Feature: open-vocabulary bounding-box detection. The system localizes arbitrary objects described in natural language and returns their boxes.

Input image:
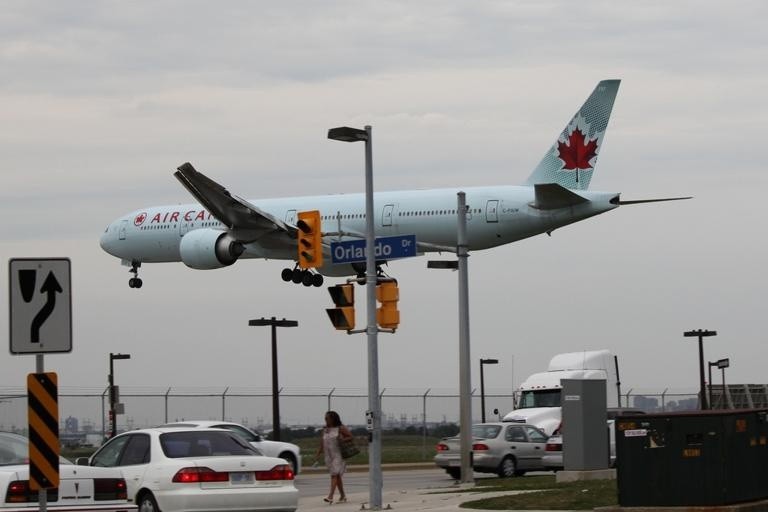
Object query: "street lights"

[328,126,382,510]
[110,353,130,437]
[248,316,298,441]
[480,359,498,423]
[684,329,729,410]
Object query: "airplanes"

[100,79,693,288]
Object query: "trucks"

[503,349,618,437]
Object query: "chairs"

[188,445,212,456]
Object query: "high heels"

[324,497,333,505]
[336,497,347,504]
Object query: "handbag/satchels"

[337,427,361,460]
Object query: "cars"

[435,408,616,480]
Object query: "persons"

[317,411,360,504]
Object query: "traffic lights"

[297,209,323,268]
[324,284,355,331]
[376,283,399,328]
